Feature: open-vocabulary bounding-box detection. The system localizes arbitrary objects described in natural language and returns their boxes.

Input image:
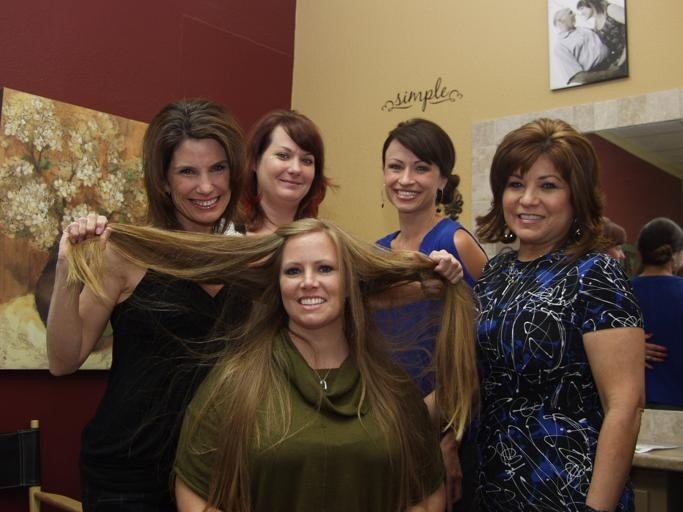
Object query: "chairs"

[0,417,82,512]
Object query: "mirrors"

[579,118,682,410]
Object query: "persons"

[553,0,628,85]
